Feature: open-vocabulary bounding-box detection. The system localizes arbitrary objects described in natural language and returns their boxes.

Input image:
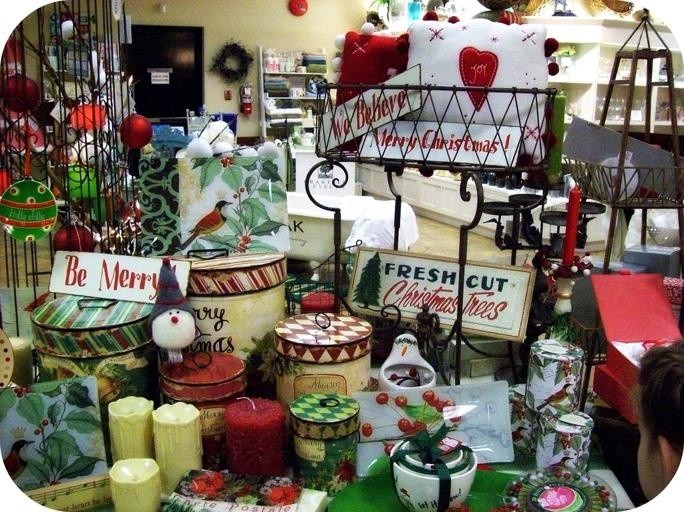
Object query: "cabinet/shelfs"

[521,14,684,137]
[257,44,328,139]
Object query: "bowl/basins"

[390,435,479,511]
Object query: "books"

[303,60,326,66]
[296,486,330,511]
[135,151,293,260]
[304,64,327,74]
[264,75,303,128]
[160,468,303,511]
[0,377,108,492]
[302,54,326,61]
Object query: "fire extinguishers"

[239,82,253,115]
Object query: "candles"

[226,396,286,474]
[562,183,581,266]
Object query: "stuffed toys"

[148,255,197,364]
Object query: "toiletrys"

[272,54,279,73]
[266,54,273,73]
[285,58,291,72]
[279,59,284,72]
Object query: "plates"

[503,465,618,511]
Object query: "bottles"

[595,150,640,203]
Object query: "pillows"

[336,31,410,151]
[407,19,551,164]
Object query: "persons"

[636,338,683,502]
